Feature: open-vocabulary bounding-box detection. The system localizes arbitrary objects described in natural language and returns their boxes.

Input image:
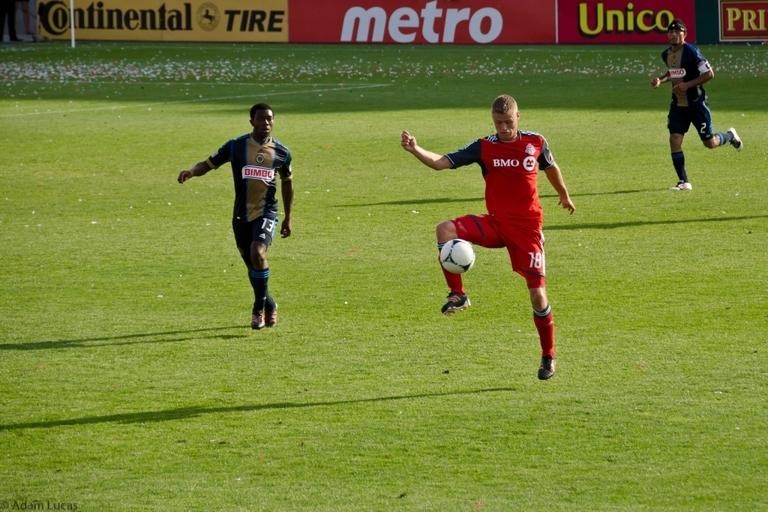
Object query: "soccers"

[439,238,475,273]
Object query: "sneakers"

[538,355,556,380]
[727,128,743,152]
[671,180,692,191]
[441,292,472,315]
[251,308,265,330]
[265,302,278,327]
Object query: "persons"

[651,20,743,191]
[401,95,576,379]
[178,103,293,329]
